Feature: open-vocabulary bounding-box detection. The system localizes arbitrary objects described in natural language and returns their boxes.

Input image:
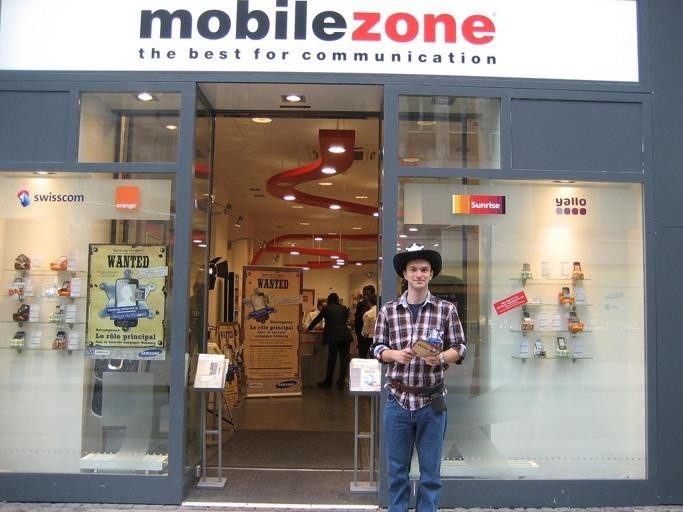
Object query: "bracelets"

[437,352,448,367]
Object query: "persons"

[369,242,468,512]
[354,285,376,359]
[304,292,355,390]
[361,295,376,359]
[303,299,326,331]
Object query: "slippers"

[559,286,574,304]
[520,337,568,358]
[521,263,532,286]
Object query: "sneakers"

[317,382,344,388]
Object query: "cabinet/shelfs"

[0,264,87,355]
[509,275,593,362]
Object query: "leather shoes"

[568,312,583,334]
[571,262,583,281]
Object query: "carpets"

[185,428,379,505]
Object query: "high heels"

[520,312,533,331]
[49,257,71,349]
[8,253,29,348]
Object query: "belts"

[390,382,443,394]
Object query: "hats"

[392,243,441,278]
